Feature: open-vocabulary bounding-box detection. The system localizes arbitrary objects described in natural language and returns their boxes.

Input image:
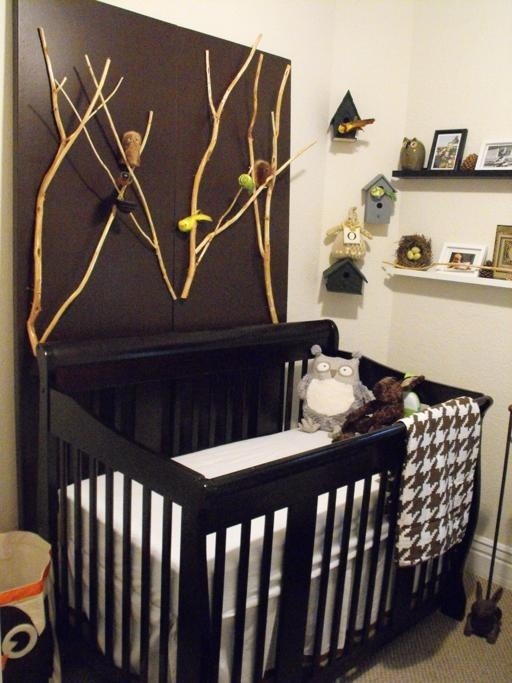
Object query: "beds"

[36,319,494,682]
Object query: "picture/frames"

[428,128,468,171]
[492,224,512,281]
[475,141,512,170]
[437,243,488,276]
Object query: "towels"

[395,395,481,567]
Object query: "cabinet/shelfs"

[387,169,512,291]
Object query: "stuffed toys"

[331,374,424,445]
[296,342,376,437]
[322,203,374,271]
[399,136,425,171]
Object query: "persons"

[449,252,462,270]
[438,140,456,167]
[494,146,512,165]
